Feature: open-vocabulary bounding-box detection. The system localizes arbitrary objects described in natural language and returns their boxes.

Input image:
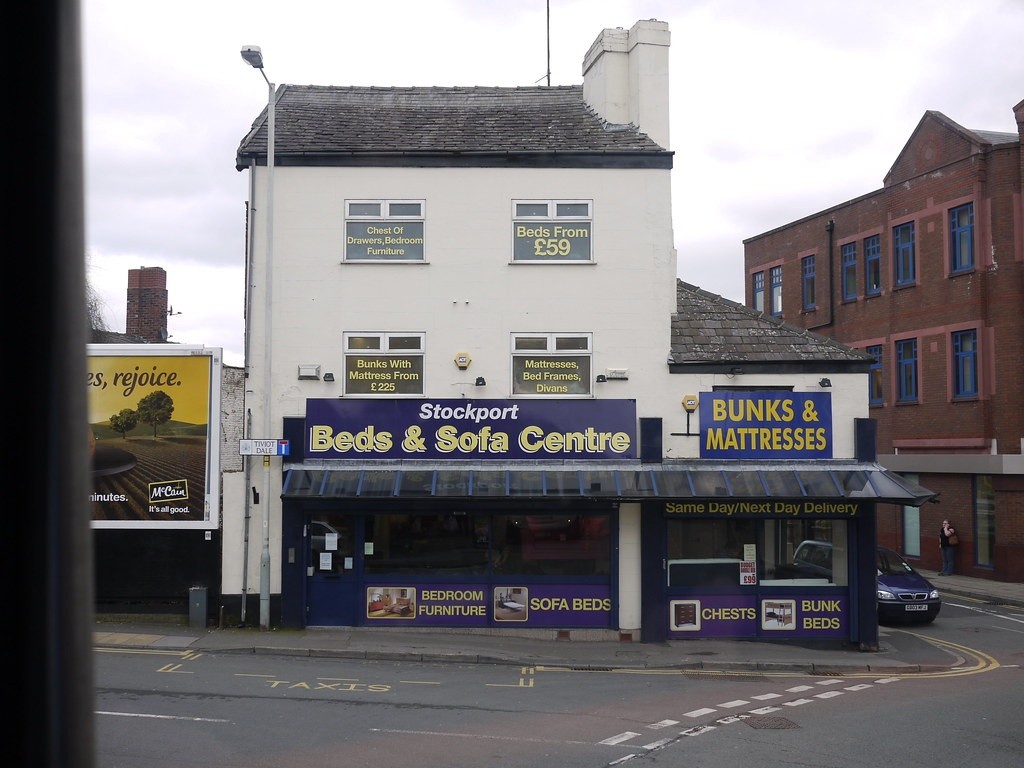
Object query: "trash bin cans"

[189,588,209,630]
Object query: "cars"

[309,520,344,570]
[792,540,942,628]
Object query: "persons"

[938,519,956,576]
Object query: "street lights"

[241,44,273,634]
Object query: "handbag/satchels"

[948,528,959,545]
